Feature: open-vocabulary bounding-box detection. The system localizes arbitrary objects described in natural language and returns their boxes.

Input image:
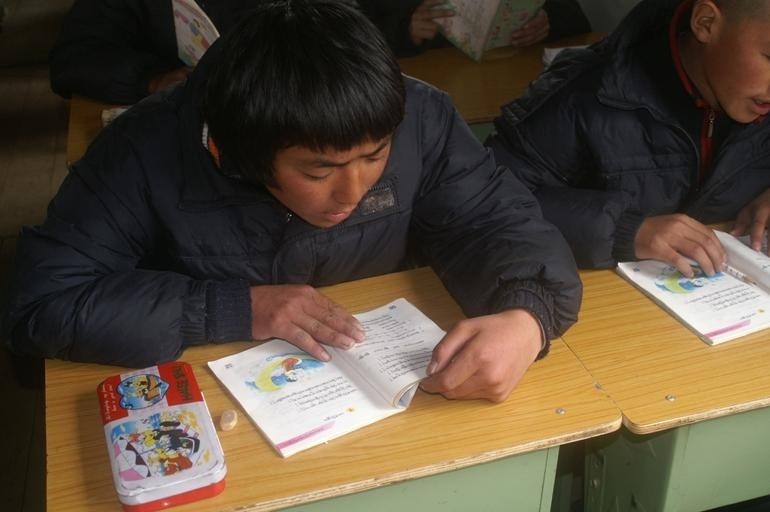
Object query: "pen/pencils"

[722,263,758,286]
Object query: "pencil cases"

[97,362,227,512]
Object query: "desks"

[45,264,624,512]
[399,25,600,143]
[563,220,768,511]
[63,94,132,169]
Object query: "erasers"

[220,410,238,432]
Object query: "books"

[614,227,770,347]
[207,296,449,459]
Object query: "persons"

[487,0,770,277]
[1,1,583,405]
[381,0,591,63]
[49,0,252,105]
